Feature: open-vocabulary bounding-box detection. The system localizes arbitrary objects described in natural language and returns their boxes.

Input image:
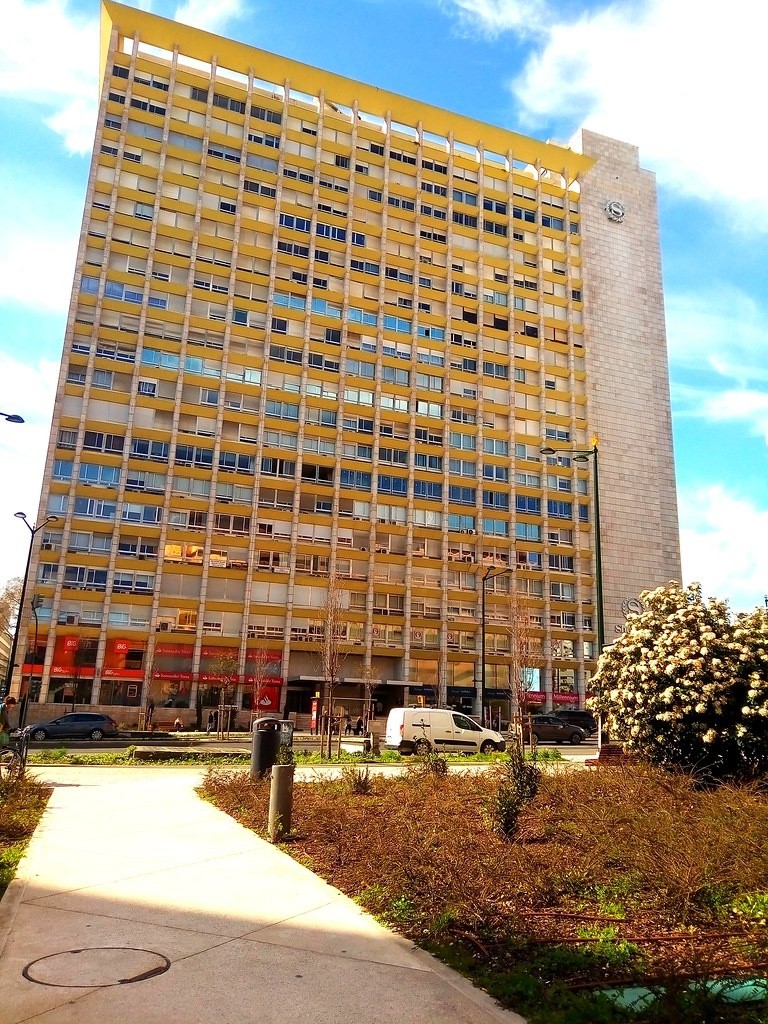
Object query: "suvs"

[541,708,598,735]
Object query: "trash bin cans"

[250,717,281,778]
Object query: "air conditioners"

[464,556,475,564]
[390,520,400,525]
[381,548,389,554]
[448,555,455,561]
[296,635,304,641]
[138,554,146,560]
[65,613,81,626]
[459,528,476,535]
[516,563,532,570]
[380,517,388,525]
[249,632,257,638]
[45,542,54,549]
[160,621,172,633]
[307,636,318,642]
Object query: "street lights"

[538,444,609,746]
[3,511,58,699]
[482,566,514,728]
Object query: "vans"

[383,705,506,758]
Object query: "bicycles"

[0,727,31,786]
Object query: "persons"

[207,710,221,732]
[356,716,363,729]
[344,716,352,735]
[0,696,17,734]
[174,716,183,732]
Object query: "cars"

[520,714,589,745]
[23,711,119,741]
